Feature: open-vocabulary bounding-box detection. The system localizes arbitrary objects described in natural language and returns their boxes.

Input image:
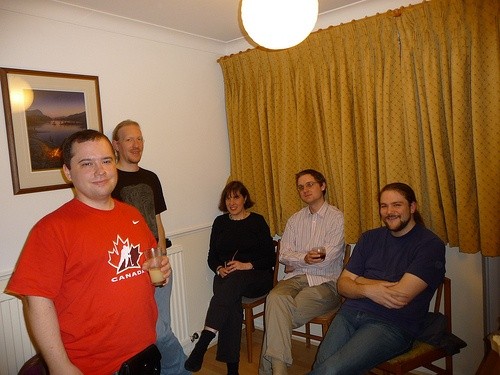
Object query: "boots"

[184,329,216,374]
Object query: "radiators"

[0,244,202,375]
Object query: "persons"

[184,181,275,375]
[259,169,346,375]
[306,182,446,375]
[4,129,171,375]
[111,119,189,375]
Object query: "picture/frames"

[0,67,104,195]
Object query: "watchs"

[217,267,223,275]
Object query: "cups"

[143,246,166,286]
[312,247,326,261]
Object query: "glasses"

[297,181,320,191]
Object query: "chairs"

[365,276,468,375]
[241,238,282,364]
[292,244,351,341]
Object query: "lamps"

[240,0,319,50]
